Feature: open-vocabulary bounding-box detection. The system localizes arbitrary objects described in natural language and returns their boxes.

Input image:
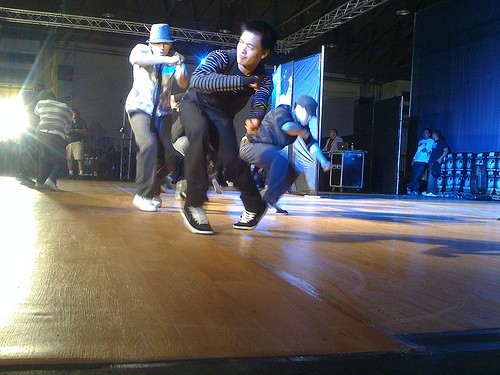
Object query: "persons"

[126,24,191,212]
[421,130,448,196]
[15,81,45,186]
[35,90,74,191]
[180,18,275,235]
[322,128,345,150]
[64,108,87,179]
[407,129,435,194]
[171,100,223,198]
[243,96,332,215]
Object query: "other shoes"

[45,178,59,191]
[79,175,85,179]
[17,175,36,185]
[212,179,221,194]
[407,187,420,196]
[421,190,437,197]
[38,177,44,186]
[67,176,76,180]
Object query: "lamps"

[325,28,337,48]
[216,8,232,33]
[395,0,410,16]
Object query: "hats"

[298,96,318,117]
[145,23,176,43]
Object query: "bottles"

[351,143,353,150]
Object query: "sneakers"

[151,193,162,208]
[234,203,269,229]
[267,203,289,215]
[182,206,213,234]
[133,192,157,212]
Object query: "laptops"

[337,142,350,150]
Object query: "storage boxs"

[329,152,365,189]
